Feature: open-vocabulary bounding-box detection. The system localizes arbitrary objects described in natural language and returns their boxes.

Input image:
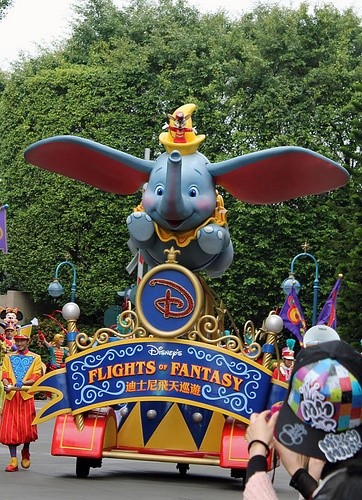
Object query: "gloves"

[38,330,45,340]
[15,381,24,388]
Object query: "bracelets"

[248,439,270,457]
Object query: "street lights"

[48,260,77,302]
[280,252,319,327]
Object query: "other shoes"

[6,457,18,471]
[21,447,30,468]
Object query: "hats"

[54,334,63,344]
[5,322,14,332]
[274,340,362,463]
[282,339,293,355]
[284,339,296,360]
[14,325,31,339]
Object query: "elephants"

[24,134,350,278]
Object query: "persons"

[38,329,71,398]
[0,324,45,472]
[242,325,362,500]
[0,307,23,328]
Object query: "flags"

[279,286,306,349]
[316,278,340,330]
